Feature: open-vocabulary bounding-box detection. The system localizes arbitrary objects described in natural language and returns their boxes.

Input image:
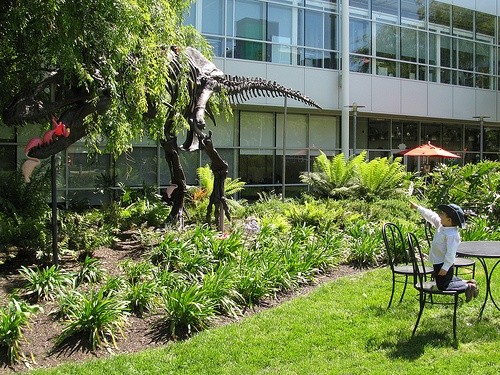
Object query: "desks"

[456,241,500,324]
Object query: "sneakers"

[468,279,479,299]
[465,284,476,302]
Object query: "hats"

[437,204,464,228]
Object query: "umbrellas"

[399,141,460,194]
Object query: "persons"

[410,201,479,302]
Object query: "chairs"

[378,223,475,339]
